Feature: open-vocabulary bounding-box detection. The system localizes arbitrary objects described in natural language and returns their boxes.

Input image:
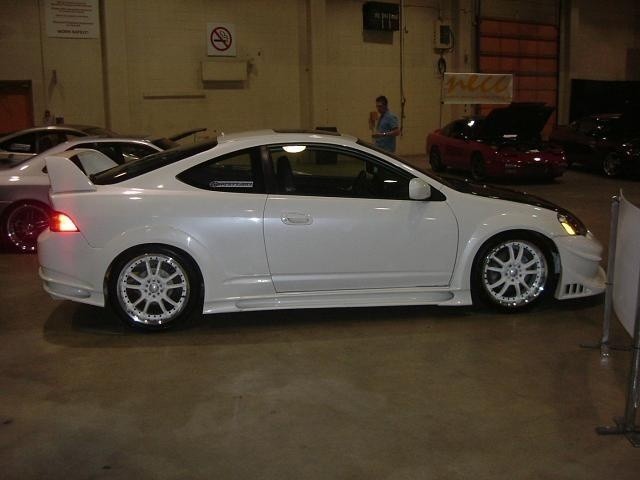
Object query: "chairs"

[276,156,303,194]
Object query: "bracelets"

[383,131,388,137]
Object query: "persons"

[368,94,401,153]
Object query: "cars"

[37,127,607,331]
[552,111,638,178]
[1,124,180,252]
[426,102,570,183]
